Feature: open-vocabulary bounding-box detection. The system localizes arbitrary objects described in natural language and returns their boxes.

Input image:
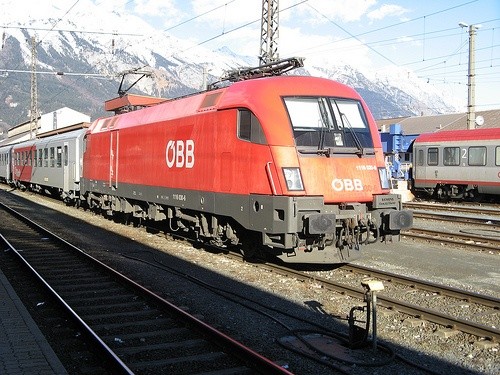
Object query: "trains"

[0,74,413,263]
[378,123,500,202]
